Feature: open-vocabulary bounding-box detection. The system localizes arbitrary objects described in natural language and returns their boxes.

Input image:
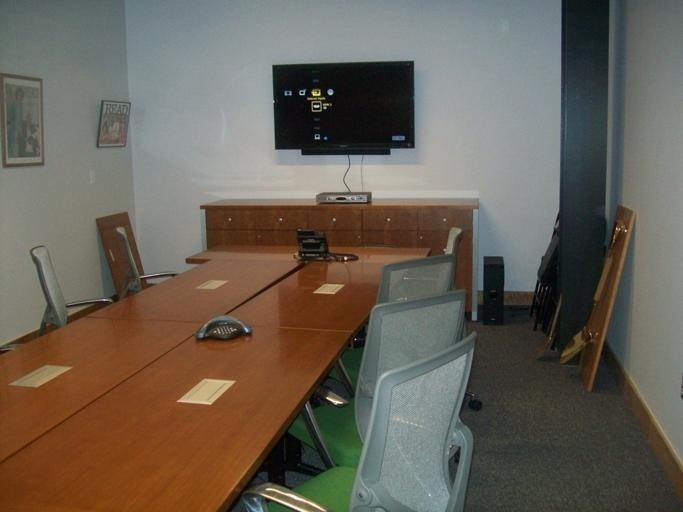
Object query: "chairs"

[443,226,463,254]
[238,328,479,512]
[96,212,178,300]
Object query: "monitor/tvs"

[272,60,415,156]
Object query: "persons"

[8,86,28,157]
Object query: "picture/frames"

[0,73,44,168]
[96,99,132,147]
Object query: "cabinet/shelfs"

[199,197,477,326]
